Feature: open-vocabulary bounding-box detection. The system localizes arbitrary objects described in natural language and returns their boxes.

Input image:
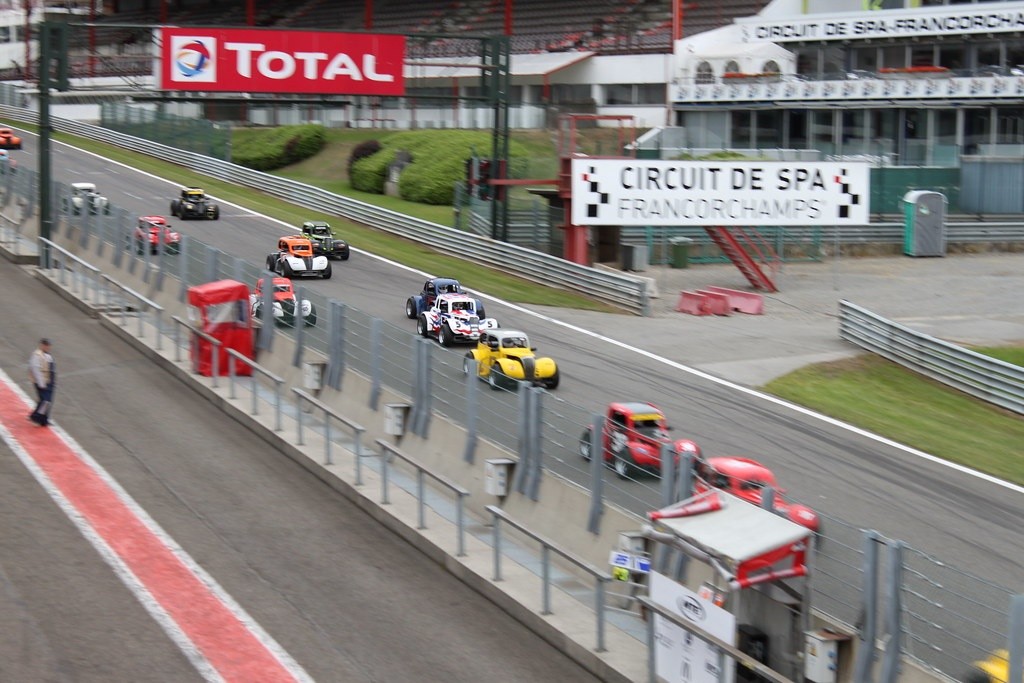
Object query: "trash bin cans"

[670,237,692,268]
[620,242,633,271]
[632,244,648,273]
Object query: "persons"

[27,339,55,426]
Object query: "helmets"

[502,338,514,348]
[314,229,322,234]
[293,245,302,250]
[438,284,449,293]
[454,302,464,310]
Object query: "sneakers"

[25,416,50,429]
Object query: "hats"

[38,338,51,345]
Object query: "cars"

[688,453,820,554]
[249,278,316,328]
[126,215,180,254]
[0,149,17,175]
[62,183,110,216]
[0,128,21,149]
[266,234,331,279]
[417,292,501,346]
[298,221,349,260]
[405,278,485,320]
[463,327,560,391]
[170,186,219,220]
[579,400,702,483]
